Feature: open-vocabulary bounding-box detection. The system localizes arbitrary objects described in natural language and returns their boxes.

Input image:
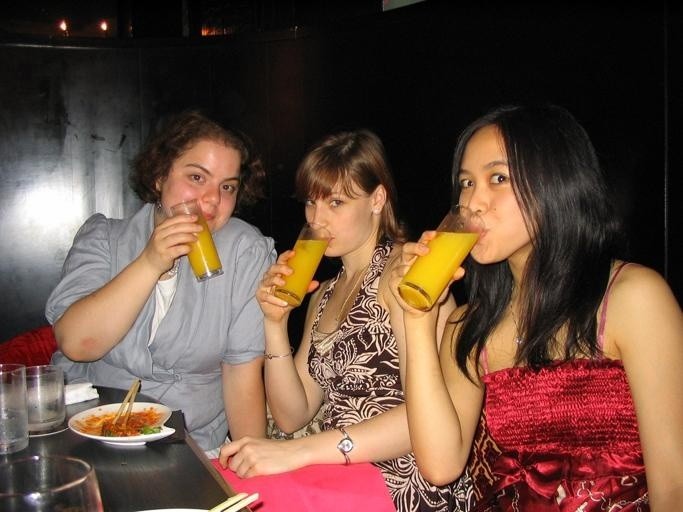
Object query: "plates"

[68,402,176,447]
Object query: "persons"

[43,107,278,451]
[388,100,683,512]
[205,129,475,512]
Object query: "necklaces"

[509,303,523,346]
[335,265,369,321]
[166,258,181,277]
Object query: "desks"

[0,379,250,511]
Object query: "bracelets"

[263,347,295,360]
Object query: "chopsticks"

[110,379,139,431]
[210,492,259,512]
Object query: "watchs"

[337,426,354,464]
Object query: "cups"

[0,364,28,454]
[1,455,103,511]
[272,221,331,308]
[171,198,226,280]
[11,366,66,431]
[397,206,483,312]
